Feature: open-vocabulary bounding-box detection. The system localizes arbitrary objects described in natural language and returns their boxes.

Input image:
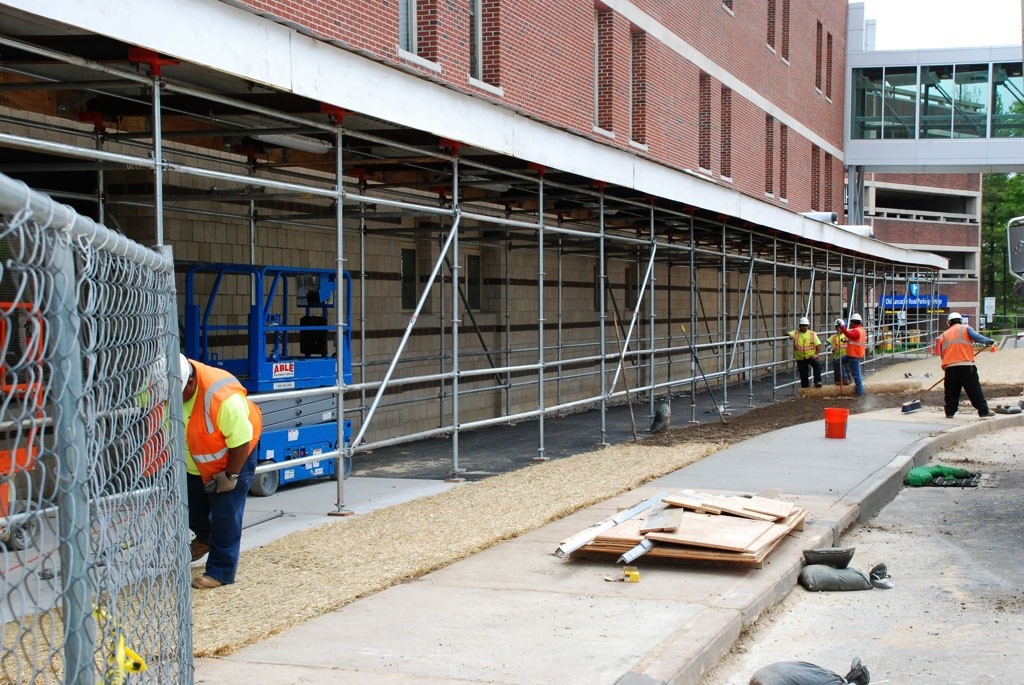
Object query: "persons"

[836,313,866,399]
[134,350,262,589]
[939,312,998,418]
[784,317,822,388]
[824,319,851,385]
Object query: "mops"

[680,324,739,428]
[823,326,852,400]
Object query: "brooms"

[900,341,994,413]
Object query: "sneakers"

[192,575,221,589]
[190,538,210,562]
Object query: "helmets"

[947,312,962,327]
[799,318,809,325]
[147,352,189,400]
[834,319,845,326]
[850,313,862,321]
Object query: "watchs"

[225,469,240,481]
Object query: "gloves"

[212,471,238,493]
[837,321,841,327]
[814,354,818,360]
[989,339,995,345]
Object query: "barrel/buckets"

[824,407,848,439]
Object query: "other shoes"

[979,411,995,417]
[816,382,822,387]
[851,394,864,400]
[947,414,953,418]
[835,382,840,385]
[843,380,849,385]
